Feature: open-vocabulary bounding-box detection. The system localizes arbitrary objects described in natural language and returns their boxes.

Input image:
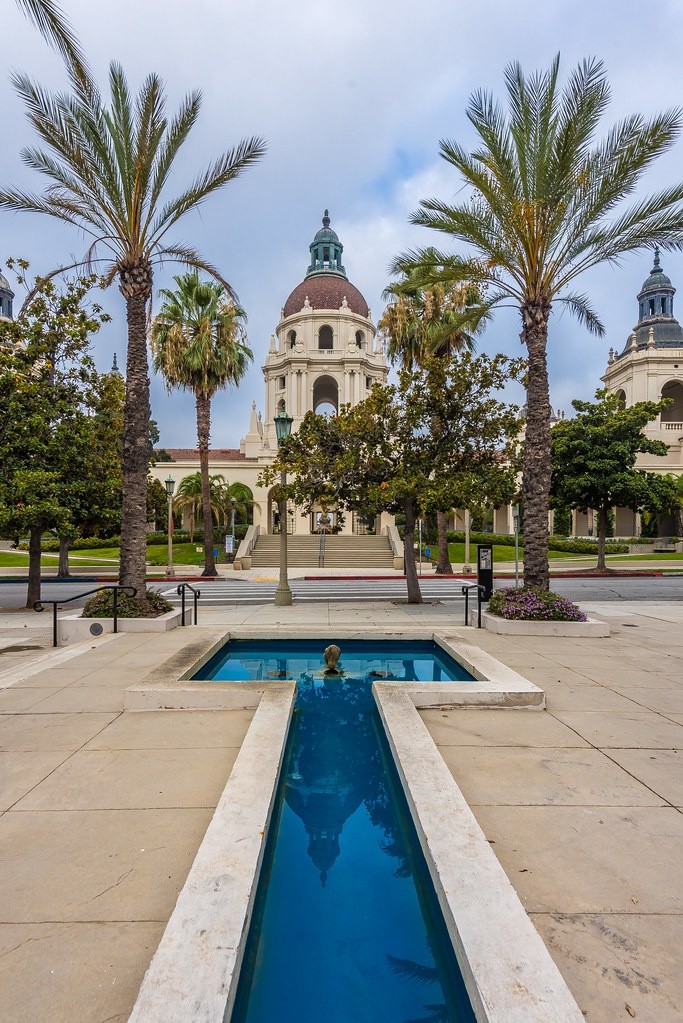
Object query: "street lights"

[271,405,293,605]
[163,473,176,578]
